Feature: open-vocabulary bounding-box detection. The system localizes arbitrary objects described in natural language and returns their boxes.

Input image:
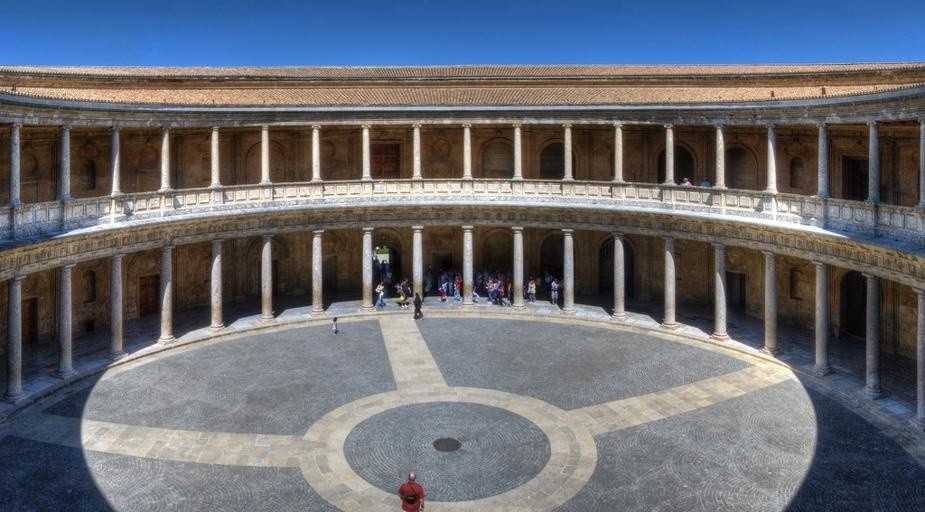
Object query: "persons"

[680,176,712,186]
[398,472,424,512]
[332,317,338,335]
[372,255,563,320]
